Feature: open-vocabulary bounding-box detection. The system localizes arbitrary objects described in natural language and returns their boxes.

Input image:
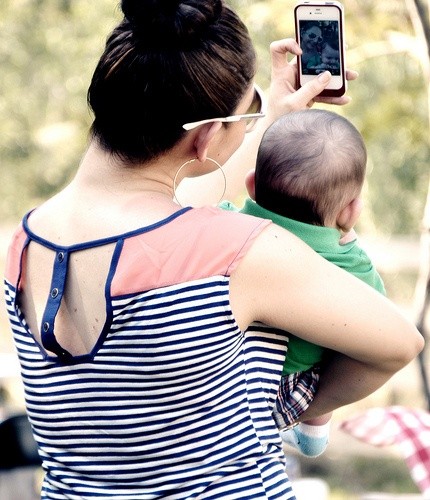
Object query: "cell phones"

[294,1,347,97]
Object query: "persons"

[218,108,387,457]
[308,38,340,69]
[1,0,425,500]
[301,22,323,70]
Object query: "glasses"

[303,30,323,41]
[183,82,266,132]
